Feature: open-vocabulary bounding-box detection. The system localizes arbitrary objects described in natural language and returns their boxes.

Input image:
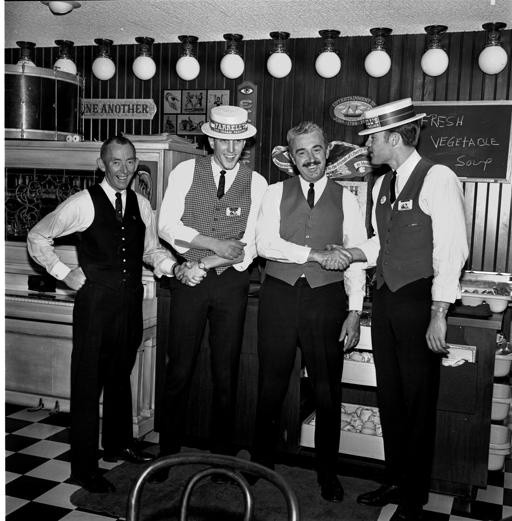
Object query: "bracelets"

[349,310,363,315]
[430,305,449,314]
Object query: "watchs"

[197,260,209,272]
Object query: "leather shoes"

[70,441,181,493]
[357,478,429,509]
[314,466,346,503]
[210,451,276,486]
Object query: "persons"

[239,119,370,502]
[321,97,469,520]
[27,135,208,495]
[164,92,225,132]
[156,103,271,483]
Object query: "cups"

[341,405,383,437]
[344,350,375,364]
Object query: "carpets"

[69,445,383,521]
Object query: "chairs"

[129,453,300,521]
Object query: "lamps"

[92,38,116,80]
[479,21,507,74]
[132,37,156,79]
[220,34,244,79]
[315,29,341,78]
[54,39,77,75]
[16,42,36,68]
[176,35,200,80]
[421,24,449,76]
[364,27,392,78]
[42,1,81,15]
[267,32,292,79]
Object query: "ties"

[306,183,316,209]
[389,170,398,204]
[217,169,226,199]
[114,192,125,221]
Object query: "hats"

[356,97,429,136]
[200,104,258,141]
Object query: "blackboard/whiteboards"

[412,100,512,184]
